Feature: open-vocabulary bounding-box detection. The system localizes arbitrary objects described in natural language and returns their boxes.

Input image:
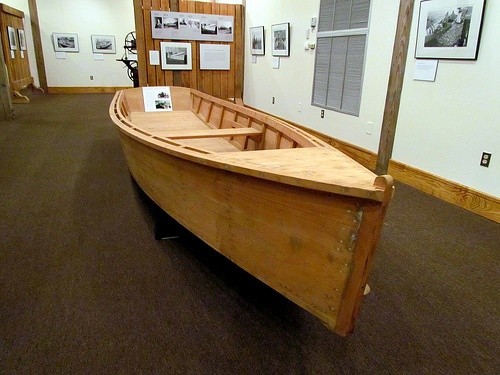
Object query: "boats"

[424,13,458,45]
[108,86,395,337]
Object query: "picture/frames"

[17,29,26,50]
[271,23,290,57]
[160,42,192,70]
[414,0,486,60]
[53,32,80,53]
[250,26,265,55]
[7,26,17,50]
[91,35,116,54]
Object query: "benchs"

[150,128,260,138]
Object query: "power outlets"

[321,110,324,118]
[480,151,492,167]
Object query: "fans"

[124,31,138,60]
